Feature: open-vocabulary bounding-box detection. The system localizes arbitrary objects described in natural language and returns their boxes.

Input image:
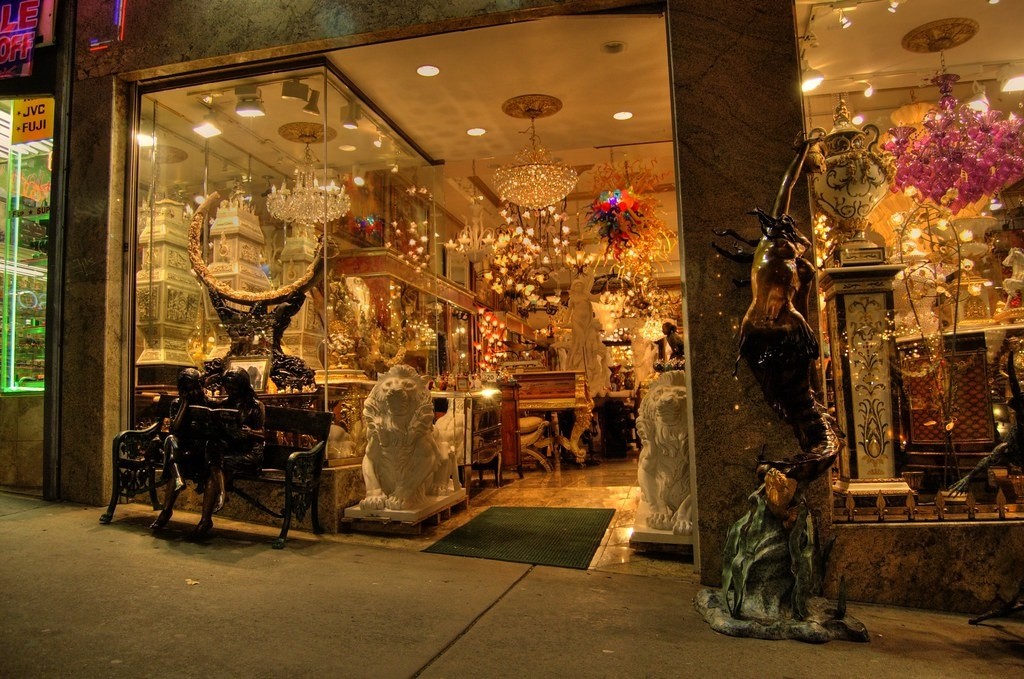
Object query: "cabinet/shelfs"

[478,382,524,483]
[1,205,49,391]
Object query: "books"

[188,405,242,437]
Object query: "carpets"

[418,505,617,570]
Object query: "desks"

[430,390,503,494]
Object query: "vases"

[608,364,621,391]
[622,369,633,388]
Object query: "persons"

[147,369,266,534]
[563,280,600,369]
[744,129,811,323]
[662,323,684,359]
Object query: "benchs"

[99,394,335,549]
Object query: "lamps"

[302,90,320,116]
[279,79,309,102]
[192,112,223,140]
[234,87,266,118]
[342,103,358,130]
[266,17,1024,373]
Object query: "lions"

[358,364,455,509]
[636,370,693,537]
